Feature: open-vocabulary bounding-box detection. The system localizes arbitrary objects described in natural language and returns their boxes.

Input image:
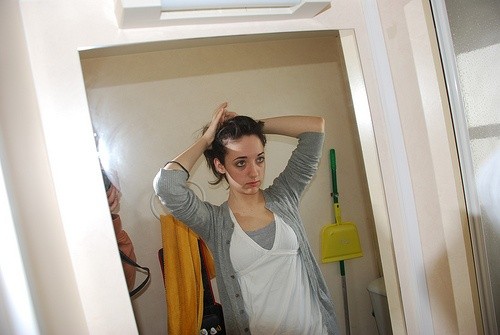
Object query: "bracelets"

[163,161,190,181]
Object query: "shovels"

[320,191,363,264]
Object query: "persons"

[153,101,340,335]
